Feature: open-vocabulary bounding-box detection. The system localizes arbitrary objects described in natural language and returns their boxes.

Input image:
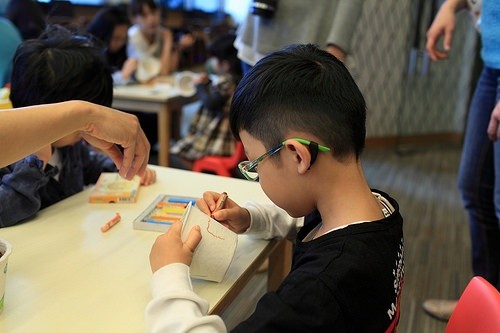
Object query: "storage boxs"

[89,172,142,203]
[133,194,200,233]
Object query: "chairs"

[193,141,245,178]
[443,276,500,333]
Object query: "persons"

[150,43,405,333]
[0,0,500,333]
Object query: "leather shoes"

[423,299,458,320]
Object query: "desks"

[1,164,305,333]
[111,73,222,167]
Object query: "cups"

[179,200,237,283]
[134,55,162,84]
[0,239,12,314]
[174,71,196,97]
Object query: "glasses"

[238,138,330,182]
